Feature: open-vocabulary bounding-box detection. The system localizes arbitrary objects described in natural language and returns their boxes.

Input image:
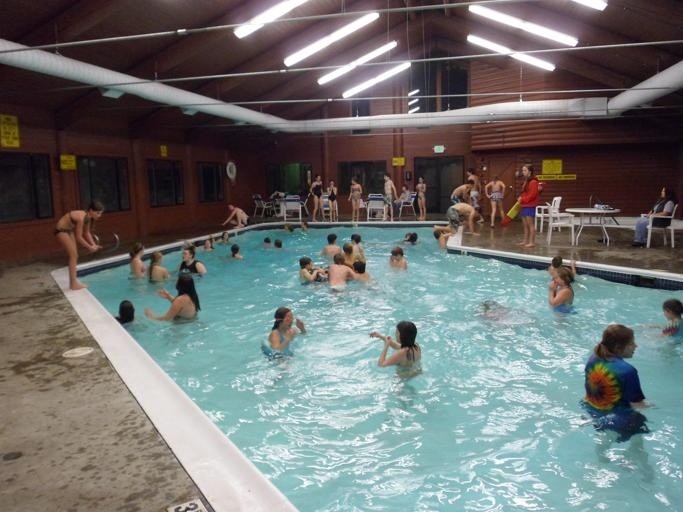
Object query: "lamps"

[465,0,609,71]
[232,0,413,100]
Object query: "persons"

[645,298,683,344]
[177,247,207,274]
[549,267,574,314]
[114,300,135,325]
[261,307,306,362]
[128,242,147,278]
[632,186,676,247]
[370,320,422,377]
[483,300,510,320]
[146,251,170,282]
[54,200,104,291]
[549,255,577,281]
[144,271,201,323]
[578,324,654,483]
[515,163,540,248]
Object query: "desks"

[563,207,622,247]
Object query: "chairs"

[545,202,577,246]
[250,189,419,223]
[640,202,679,249]
[534,195,562,233]
